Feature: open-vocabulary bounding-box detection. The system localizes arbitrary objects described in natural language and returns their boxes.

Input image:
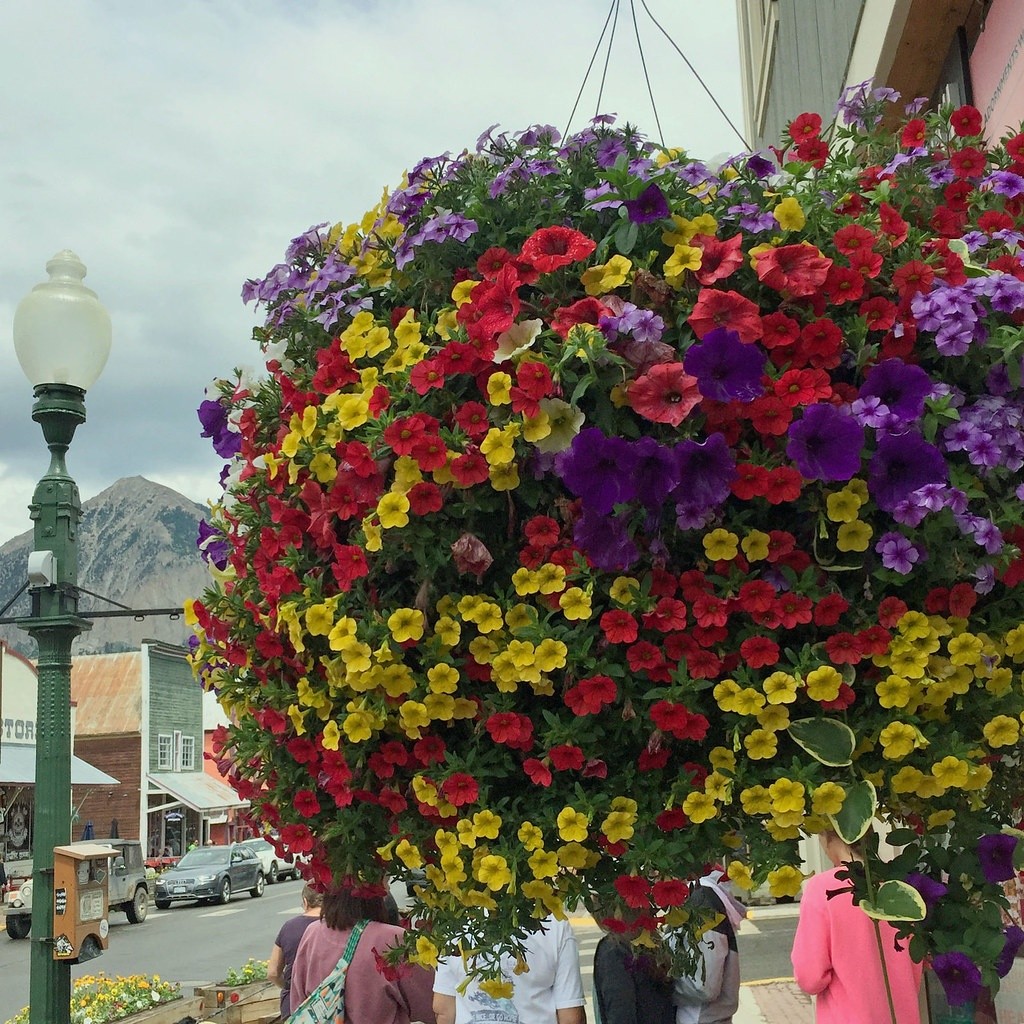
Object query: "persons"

[790,828,924,1023]
[265,872,586,1023]
[592,863,741,1024]
[0,853,10,902]
[187,839,217,852]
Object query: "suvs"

[3,838,149,939]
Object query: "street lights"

[0,251,188,1024]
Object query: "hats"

[207,840,213,844]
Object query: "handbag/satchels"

[284,919,370,1024]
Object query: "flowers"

[226,956,270,986]
[4,969,184,1024]
[180,76,1024,1010]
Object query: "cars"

[242,836,309,884]
[153,842,264,909]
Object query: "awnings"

[0,742,123,785]
[146,772,251,813]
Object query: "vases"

[112,996,204,1024]
[193,982,282,1024]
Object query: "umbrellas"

[81,821,95,841]
[110,818,120,839]
